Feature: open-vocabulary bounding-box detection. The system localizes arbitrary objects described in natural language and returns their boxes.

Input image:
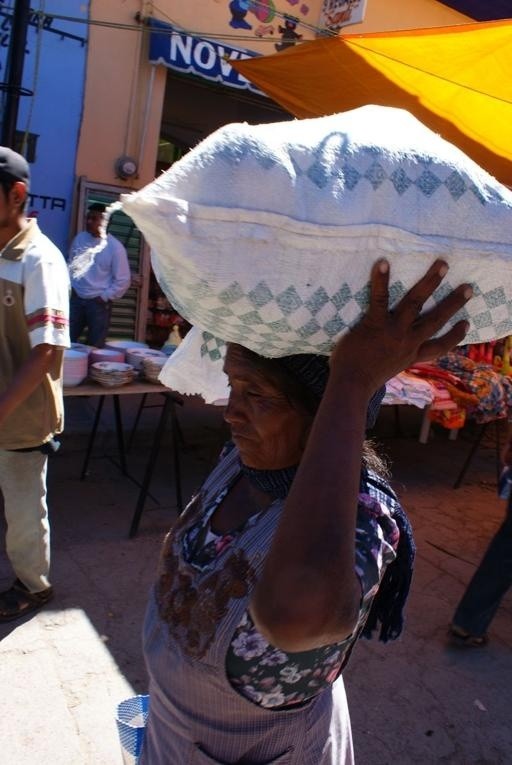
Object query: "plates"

[58,335,188,390]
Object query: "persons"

[449,492,510,650]
[0,142,71,626]
[68,202,133,345]
[136,255,475,765]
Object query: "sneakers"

[0,575,55,625]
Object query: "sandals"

[445,621,493,650]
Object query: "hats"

[0,143,32,189]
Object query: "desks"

[59,377,191,538]
[379,393,505,498]
